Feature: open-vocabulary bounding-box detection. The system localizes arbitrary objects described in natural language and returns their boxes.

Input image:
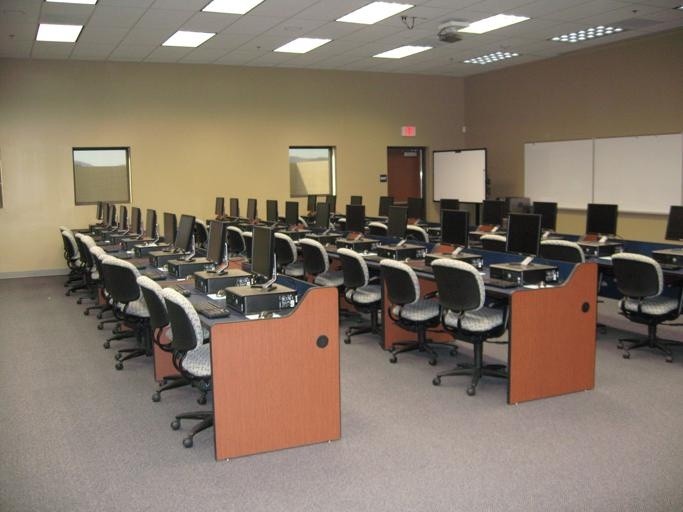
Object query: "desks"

[149,267,341,464]
[381,245,599,403]
[216,216,438,352]
[428,220,682,313]
[67,225,227,381]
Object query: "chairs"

[338,218,347,231]
[194,220,208,250]
[540,240,585,263]
[298,238,365,325]
[406,225,429,243]
[136,277,210,402]
[97,255,152,370]
[297,217,308,228]
[226,227,245,258]
[76,236,97,305]
[83,247,113,320]
[611,253,679,364]
[336,249,382,344]
[58,226,70,260]
[379,259,460,366]
[480,234,507,253]
[242,231,253,264]
[432,258,506,397]
[369,221,388,236]
[161,286,214,448]
[62,231,81,296]
[273,232,304,277]
[74,233,85,298]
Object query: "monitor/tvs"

[407,198,425,224]
[207,220,229,275]
[326,196,336,218]
[110,205,118,231]
[440,199,459,210]
[440,209,468,257]
[230,199,240,222]
[162,213,177,252]
[216,198,225,221]
[316,203,331,235]
[483,200,504,233]
[252,226,277,291]
[286,202,298,230]
[388,206,407,248]
[247,199,258,224]
[351,196,362,205]
[132,207,144,239]
[267,200,280,227]
[346,205,365,241]
[120,206,130,238]
[97,202,102,219]
[533,202,556,229]
[103,203,110,225]
[585,204,617,243]
[176,214,196,261]
[307,195,317,216]
[506,213,542,268]
[147,209,160,246]
[663,206,682,249]
[379,197,393,224]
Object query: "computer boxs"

[90,224,104,233]
[112,234,129,245]
[122,240,144,250]
[651,248,683,265]
[490,262,559,286]
[425,252,483,270]
[337,237,381,253]
[577,240,624,257]
[150,251,187,267]
[168,257,215,278]
[378,242,425,261]
[306,233,343,247]
[101,231,116,239]
[194,269,252,294]
[96,227,104,234]
[225,283,297,315]
[279,229,312,241]
[135,244,162,257]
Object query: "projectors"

[439,33,463,43]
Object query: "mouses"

[183,289,191,297]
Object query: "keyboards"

[484,278,518,289]
[193,301,231,319]
[163,284,186,294]
[145,266,167,280]
[660,263,680,270]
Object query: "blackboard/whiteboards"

[523,134,683,216]
[432,147,487,205]
